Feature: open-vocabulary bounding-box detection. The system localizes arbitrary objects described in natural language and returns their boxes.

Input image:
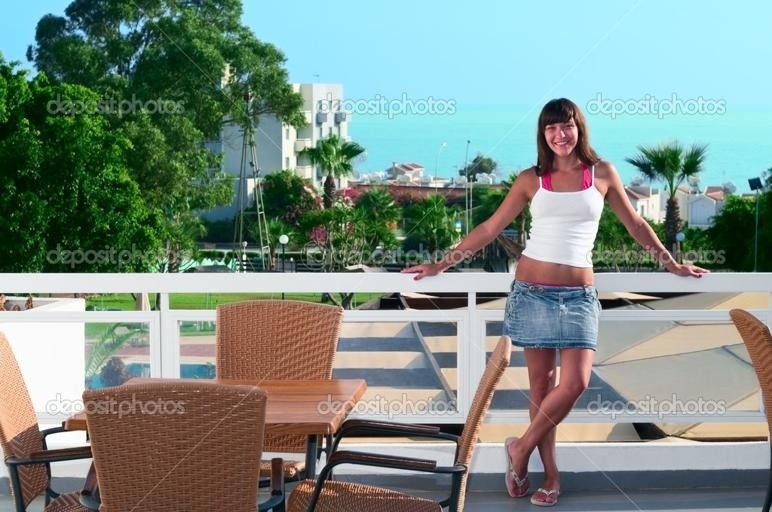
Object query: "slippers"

[530,487,561,507]
[504,437,530,497]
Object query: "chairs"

[0,300,514,512]
[730,308,772,511]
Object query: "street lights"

[676,232,685,264]
[748,178,763,272]
[279,235,289,299]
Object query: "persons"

[400,98,711,507]
